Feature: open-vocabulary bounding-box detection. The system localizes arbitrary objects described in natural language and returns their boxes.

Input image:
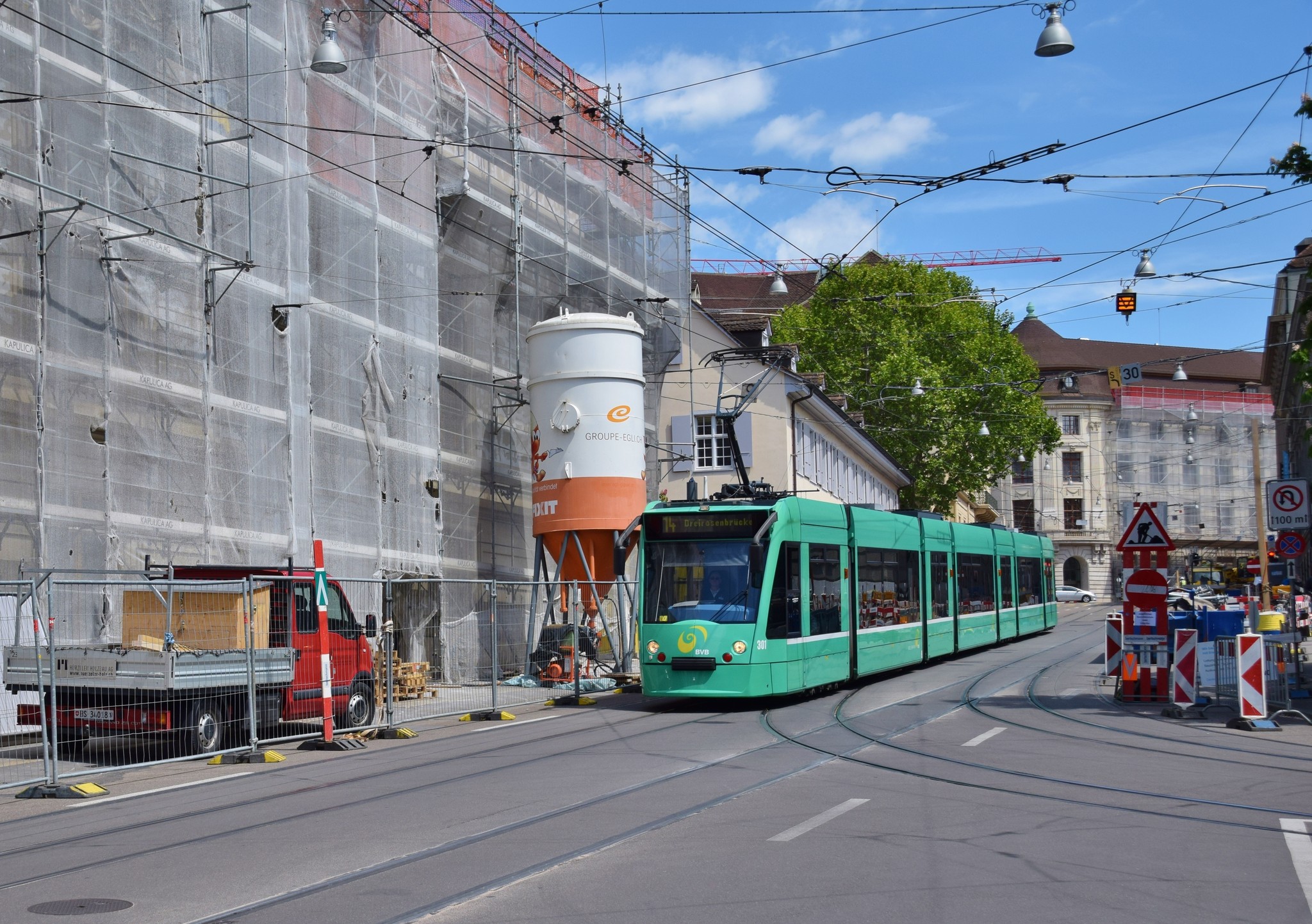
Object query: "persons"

[700,570,732,605]
[1294,573,1304,596]
[1179,577,1187,585]
[1224,568,1246,588]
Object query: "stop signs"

[1247,559,1261,573]
[1123,569,1169,610]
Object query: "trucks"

[0,554,381,756]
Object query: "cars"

[1055,585,1097,603]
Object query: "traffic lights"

[1269,551,1276,563]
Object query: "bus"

[611,344,1058,698]
[1187,547,1262,585]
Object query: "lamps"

[309,1,1197,470]
